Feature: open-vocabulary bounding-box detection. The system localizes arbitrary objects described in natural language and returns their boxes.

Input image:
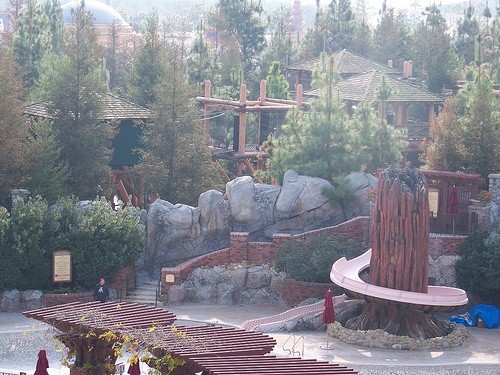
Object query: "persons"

[93,277,109,303]
